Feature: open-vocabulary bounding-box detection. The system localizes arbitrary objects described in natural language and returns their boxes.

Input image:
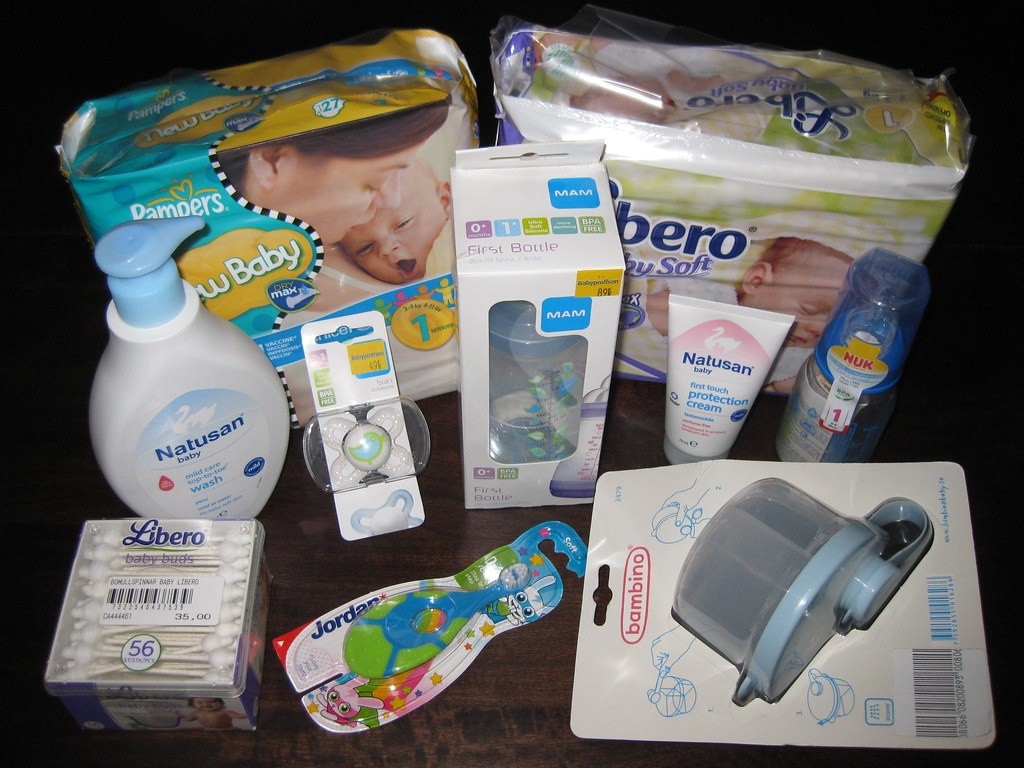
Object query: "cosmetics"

[662,293,796,465]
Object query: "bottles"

[488,301,588,464]
[775,247,930,463]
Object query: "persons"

[532,33,731,129]
[640,235,854,392]
[221,95,457,311]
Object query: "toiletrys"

[774,248,936,466]
[662,291,801,462]
[90,216,289,532]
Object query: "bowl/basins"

[671,477,933,707]
